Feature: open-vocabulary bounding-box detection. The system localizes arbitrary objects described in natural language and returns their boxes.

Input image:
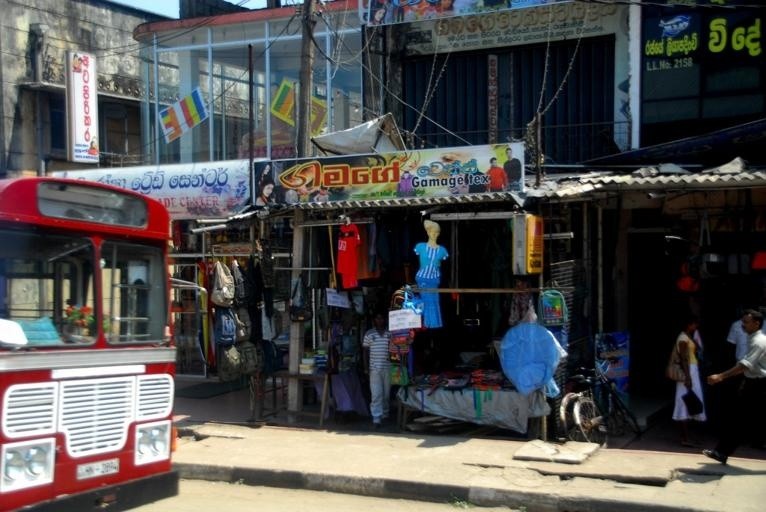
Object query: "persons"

[256,179,276,207]
[413,219,450,328]
[487,157,508,191]
[370,2,387,26]
[361,311,393,430]
[665,307,766,464]
[503,148,522,191]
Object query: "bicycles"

[558,372,626,442]
[571,358,648,444]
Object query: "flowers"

[62,304,110,333]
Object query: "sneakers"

[371,417,394,433]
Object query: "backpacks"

[232,261,256,307]
[237,341,260,383]
[216,345,243,384]
[209,259,238,310]
[539,288,570,327]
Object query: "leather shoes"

[702,448,728,465]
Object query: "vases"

[70,325,91,336]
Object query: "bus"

[0,173,182,511]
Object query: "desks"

[395,382,553,439]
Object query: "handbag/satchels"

[287,273,313,322]
[663,361,689,382]
[233,304,253,343]
[214,306,238,347]
[681,387,704,416]
